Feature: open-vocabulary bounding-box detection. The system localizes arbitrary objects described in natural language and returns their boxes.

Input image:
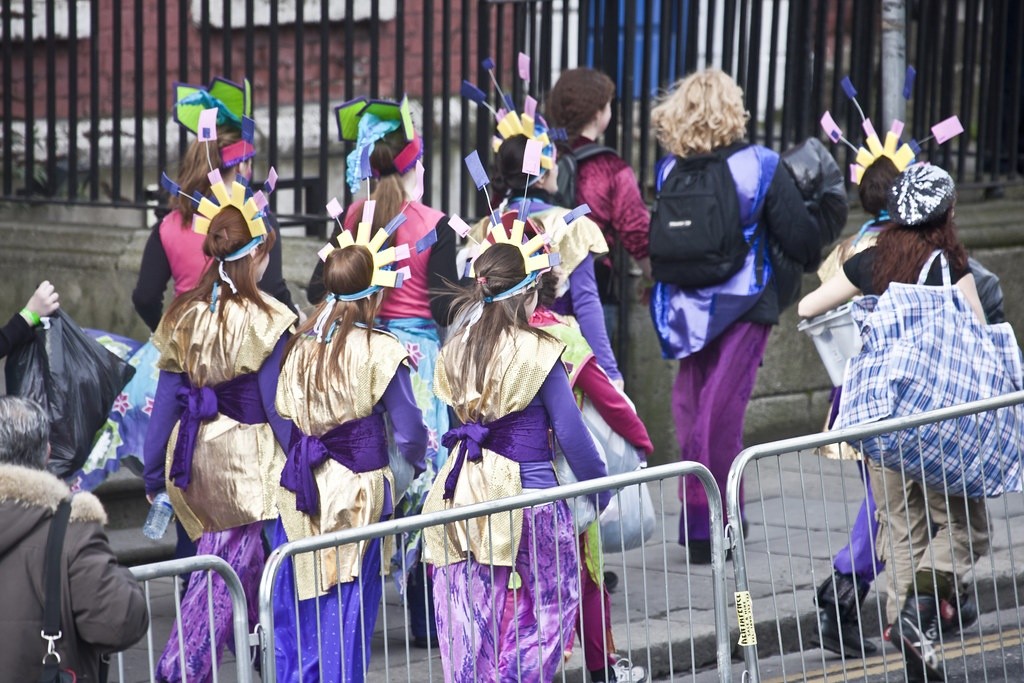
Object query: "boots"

[943,603,981,636]
[886,571,954,683]
[810,572,879,657]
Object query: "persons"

[132,50,647,683]
[646,71,817,563]
[797,66,1005,678]
[0,396,149,683]
[0,279,60,360]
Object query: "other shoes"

[603,572,618,591]
[688,542,737,564]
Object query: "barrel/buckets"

[796,301,861,387]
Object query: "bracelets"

[21,308,39,325]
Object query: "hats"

[886,162,954,229]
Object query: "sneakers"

[595,658,647,683]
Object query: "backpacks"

[555,140,613,209]
[647,141,762,290]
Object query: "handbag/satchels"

[839,250,1024,497]
[7,306,134,476]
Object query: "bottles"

[142,488,173,541]
